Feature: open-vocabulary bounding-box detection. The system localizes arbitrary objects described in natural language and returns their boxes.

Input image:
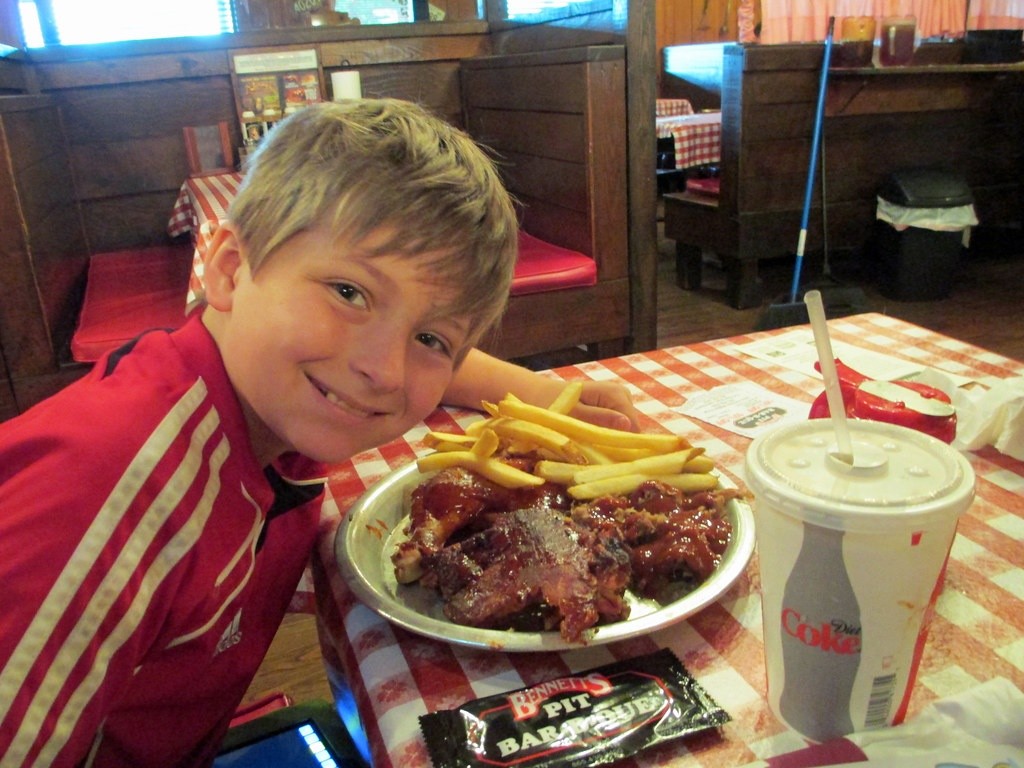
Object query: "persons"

[0,93,641,768]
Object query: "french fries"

[416,375,719,500]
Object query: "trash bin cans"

[872,166,975,302]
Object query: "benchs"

[0,43,629,411]
[664,39,967,289]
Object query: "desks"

[657,98,694,120]
[657,114,725,170]
[165,171,245,316]
[321,313,1024,767]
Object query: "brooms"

[755,17,856,332]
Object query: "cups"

[840,17,877,67]
[745,359,976,744]
[879,15,917,66]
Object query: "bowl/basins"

[968,29,1023,65]
[920,37,964,65]
[334,451,755,652]
